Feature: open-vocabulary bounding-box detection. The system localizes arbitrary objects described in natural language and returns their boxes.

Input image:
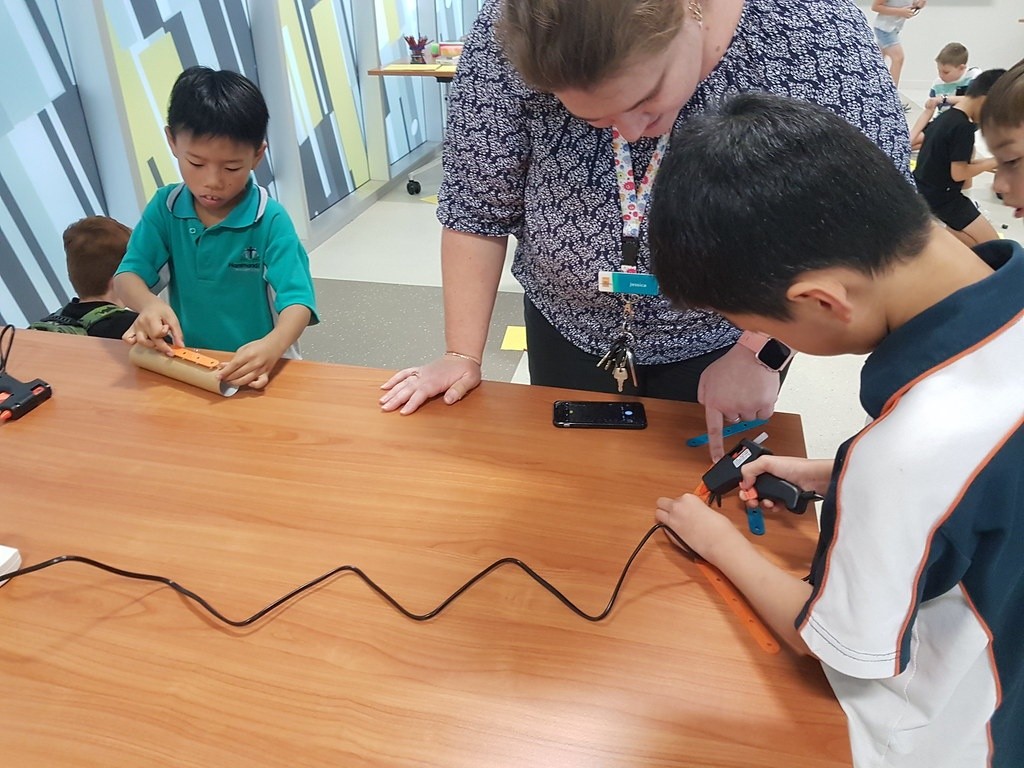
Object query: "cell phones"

[552,398,648,431]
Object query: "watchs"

[736,329,795,372]
[941,94,948,104]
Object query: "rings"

[725,417,740,423]
[411,372,420,378]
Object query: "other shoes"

[902,103,911,111]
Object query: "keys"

[596,339,638,393]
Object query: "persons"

[112,66,320,389]
[870,0,1024,248]
[647,96,1024,768]
[26,216,173,345]
[378,0,920,464]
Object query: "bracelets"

[445,352,481,366]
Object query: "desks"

[1,327,855,768]
[368,56,457,195]
